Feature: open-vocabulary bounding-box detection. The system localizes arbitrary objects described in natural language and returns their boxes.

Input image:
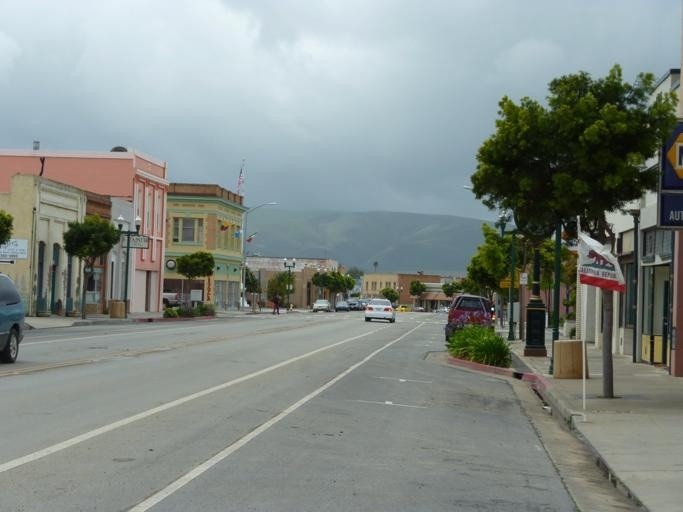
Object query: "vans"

[444,294,496,340]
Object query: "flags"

[576,233,626,292]
[237,168,245,191]
[246,232,257,243]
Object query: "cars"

[364,299,395,323]
[312,299,331,312]
[335,297,370,312]
[0,273,26,365]
[395,304,424,312]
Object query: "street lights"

[283,256,295,310]
[114,215,141,319]
[499,209,521,341]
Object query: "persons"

[272,292,283,315]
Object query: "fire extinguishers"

[55,299,63,316]
